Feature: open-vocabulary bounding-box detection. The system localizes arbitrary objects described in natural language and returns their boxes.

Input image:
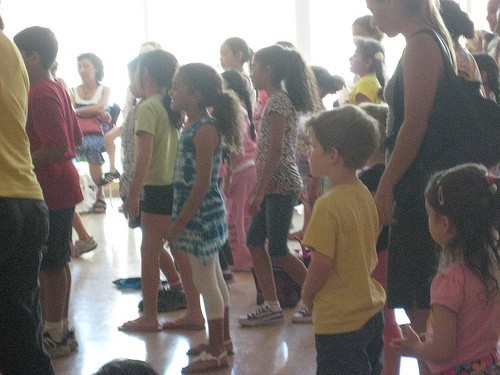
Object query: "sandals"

[180,357,231,374]
[162,321,206,330]
[186,341,235,355]
[93,199,107,213]
[118,320,163,331]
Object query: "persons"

[0,0,500,375]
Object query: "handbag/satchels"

[76,115,105,136]
[406,29,500,179]
[251,235,310,308]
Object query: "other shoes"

[222,271,235,287]
[40,326,79,360]
[137,286,188,312]
[73,236,98,256]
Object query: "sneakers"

[292,305,314,323]
[237,303,285,326]
[97,169,121,186]
